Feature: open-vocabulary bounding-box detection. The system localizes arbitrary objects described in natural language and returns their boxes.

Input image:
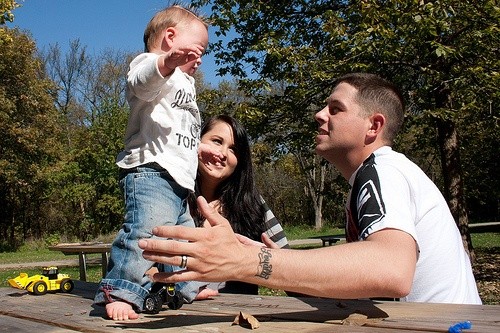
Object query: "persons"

[92,5,223,321]
[140,75,482,305]
[198,114,310,297]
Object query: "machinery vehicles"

[8,266,75,296]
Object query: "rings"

[180,256,187,268]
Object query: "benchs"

[48,238,112,281]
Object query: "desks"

[0,281,500,333]
